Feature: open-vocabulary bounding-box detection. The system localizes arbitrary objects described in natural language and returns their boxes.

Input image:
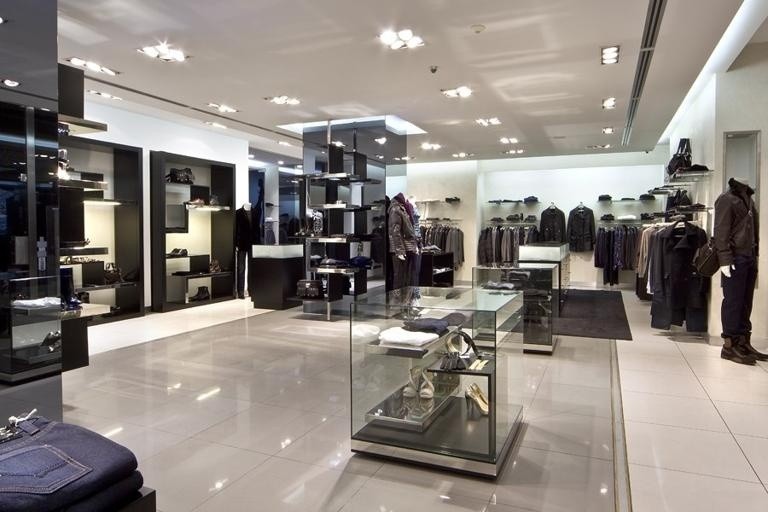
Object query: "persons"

[235,202,261,300]
[714,177,768,366]
[407,196,424,310]
[388,193,421,321]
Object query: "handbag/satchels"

[668,138,691,174]
[693,239,719,278]
[171,168,193,183]
[105,262,125,286]
[297,279,324,299]
[103,306,122,316]
[673,189,691,205]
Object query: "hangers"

[487,223,536,228]
[672,212,687,229]
[422,221,458,227]
[599,222,642,227]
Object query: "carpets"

[460,288,631,341]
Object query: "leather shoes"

[188,198,204,205]
[166,249,187,257]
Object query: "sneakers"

[737,343,768,360]
[722,344,755,364]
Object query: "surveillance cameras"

[431,66,437,73]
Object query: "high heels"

[465,383,488,415]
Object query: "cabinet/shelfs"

[59,134,234,320]
[473,264,559,356]
[350,286,525,481]
[303,123,388,313]
[415,170,714,222]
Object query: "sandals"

[403,365,435,399]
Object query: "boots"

[188,287,210,301]
[60,268,82,310]
